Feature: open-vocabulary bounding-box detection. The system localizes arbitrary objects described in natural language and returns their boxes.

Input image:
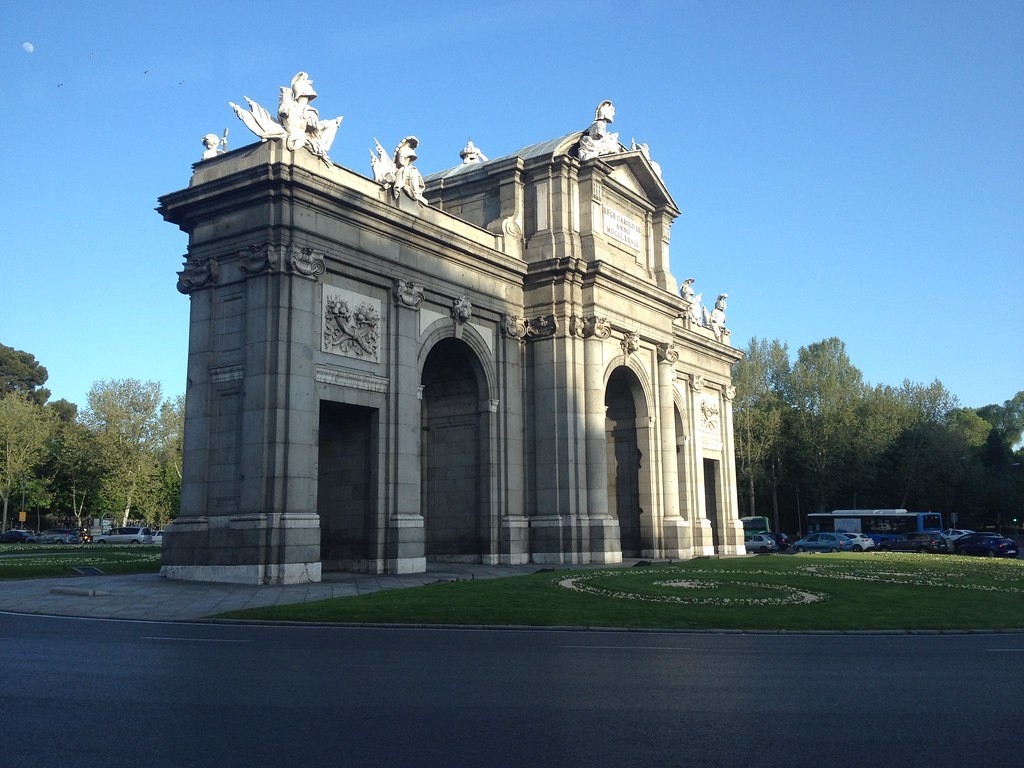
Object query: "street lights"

[19,474,26,530]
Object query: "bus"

[805,509,943,533]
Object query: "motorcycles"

[81,532,93,545]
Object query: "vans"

[740,516,772,541]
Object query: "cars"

[877,533,938,554]
[0,530,35,544]
[953,532,1019,559]
[758,531,789,552]
[917,529,976,554]
[150,530,164,546]
[744,535,776,553]
[842,533,876,552]
[793,532,853,552]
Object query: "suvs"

[26,528,79,544]
[93,527,152,544]
